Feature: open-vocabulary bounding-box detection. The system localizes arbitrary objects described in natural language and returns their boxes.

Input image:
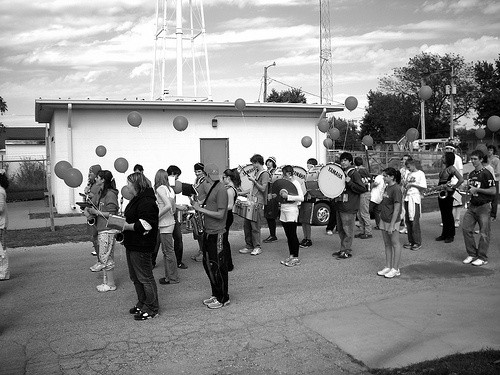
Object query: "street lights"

[264,61,276,102]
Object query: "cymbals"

[272,179,299,204]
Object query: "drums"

[308,163,347,199]
[277,165,308,196]
[236,164,257,196]
[232,195,259,222]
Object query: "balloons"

[474,115,500,140]
[127,111,142,127]
[54,161,83,188]
[114,157,128,173]
[324,139,333,148]
[173,115,188,131]
[405,128,419,143]
[362,135,374,146]
[345,96,358,111]
[476,143,487,156]
[301,136,312,148]
[96,145,106,157]
[235,98,245,111]
[418,85,433,101]
[318,121,341,140]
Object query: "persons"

[166,165,189,269]
[153,169,181,284]
[216,168,242,271]
[134,164,152,187]
[83,164,104,256]
[376,167,402,278]
[0,169,10,280]
[81,170,119,294]
[189,163,208,261]
[192,163,230,310]
[239,142,499,267]
[116,173,160,322]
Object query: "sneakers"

[208,299,230,308]
[384,268,400,278]
[203,296,218,305]
[377,267,392,276]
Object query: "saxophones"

[113,196,124,244]
[79,182,94,213]
[87,188,103,226]
[421,180,452,199]
[186,178,206,236]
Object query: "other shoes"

[129,306,140,314]
[263,236,277,242]
[97,284,116,292]
[135,310,158,321]
[178,263,188,269]
[196,252,204,262]
[92,251,98,255]
[192,250,201,260]
[332,250,342,256]
[251,247,262,255]
[299,239,308,246]
[159,278,170,284]
[280,255,294,264]
[326,218,497,266]
[336,252,351,259]
[90,262,103,271]
[285,257,301,266]
[239,248,254,253]
[301,239,312,249]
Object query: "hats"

[266,157,277,168]
[204,164,221,181]
